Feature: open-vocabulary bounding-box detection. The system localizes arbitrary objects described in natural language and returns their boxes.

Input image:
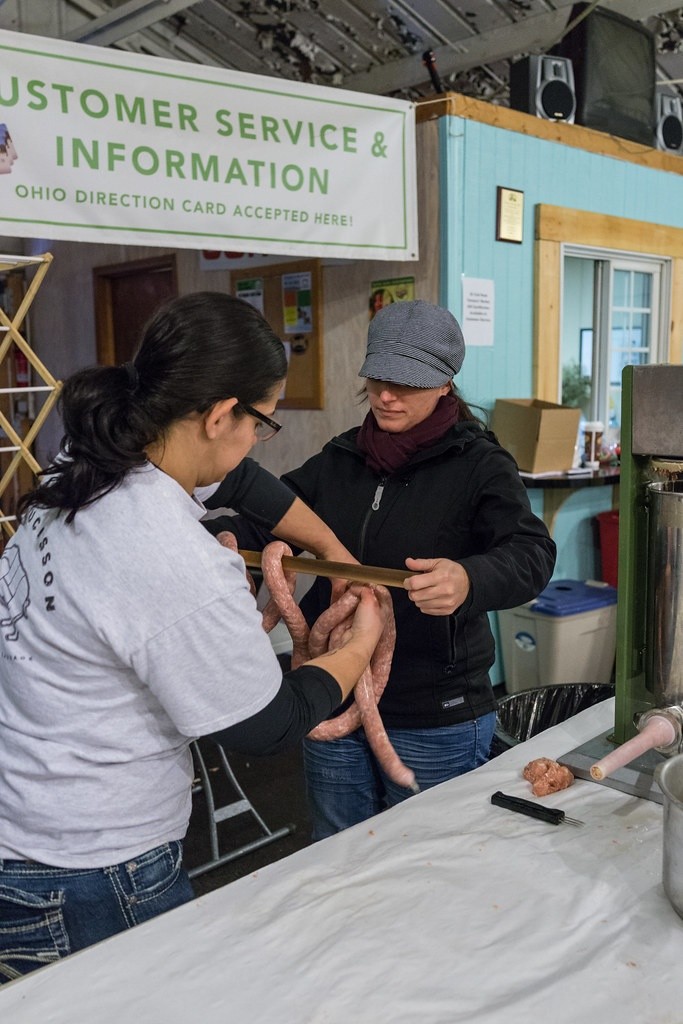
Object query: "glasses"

[198,394,283,441]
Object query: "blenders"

[556,365,683,805]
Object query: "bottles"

[608,409,620,466]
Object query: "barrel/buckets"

[654,751,683,921]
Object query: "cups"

[584,420,603,472]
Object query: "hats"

[358,300,465,388]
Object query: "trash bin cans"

[493,682,615,753]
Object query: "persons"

[200,303,557,844]
[0,290,391,988]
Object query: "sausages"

[217,531,418,791]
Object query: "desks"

[519,467,621,536]
[0,697,683,1024]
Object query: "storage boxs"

[498,578,617,694]
[488,398,581,475]
